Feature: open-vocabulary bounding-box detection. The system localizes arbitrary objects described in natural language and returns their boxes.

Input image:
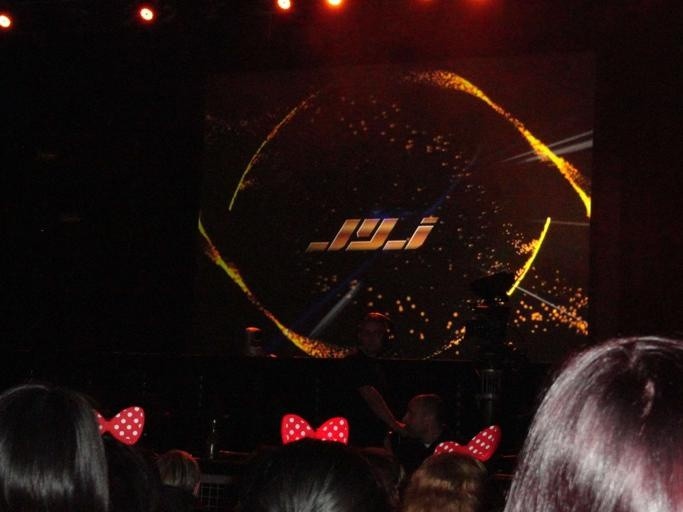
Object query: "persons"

[1,317,682,511]
